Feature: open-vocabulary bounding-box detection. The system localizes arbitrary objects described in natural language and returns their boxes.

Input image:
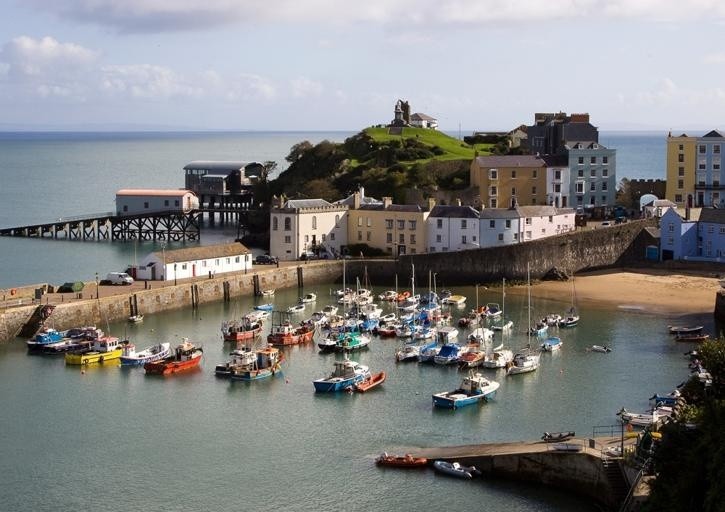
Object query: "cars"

[600,221,613,228]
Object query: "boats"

[430,367,500,409]
[434,460,481,480]
[543,427,640,460]
[617,321,714,430]
[376,453,428,468]
[314,353,369,394]
[591,345,613,355]
[346,369,387,394]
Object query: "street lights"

[304,242,308,264]
[94,271,100,298]
[618,405,626,457]
[243,250,247,273]
[239,222,247,245]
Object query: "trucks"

[106,271,134,285]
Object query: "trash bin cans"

[35,289,42,299]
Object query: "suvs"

[256,254,277,264]
[614,216,627,225]
[302,251,318,260]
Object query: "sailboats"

[317,257,582,380]
